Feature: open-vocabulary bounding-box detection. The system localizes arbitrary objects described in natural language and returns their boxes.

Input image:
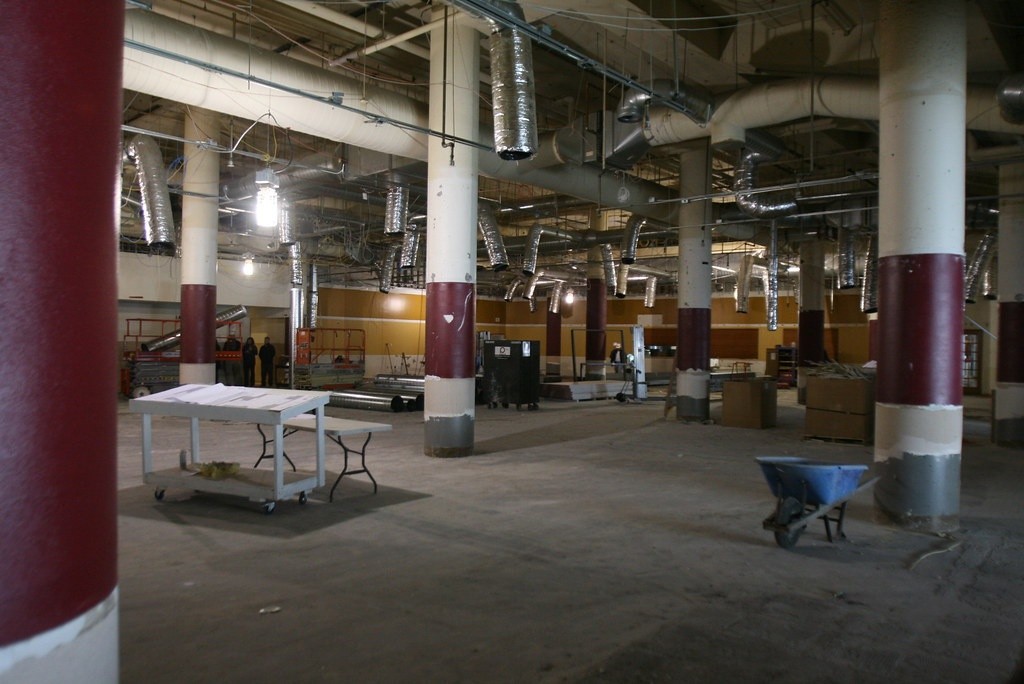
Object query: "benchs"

[255,413,393,502]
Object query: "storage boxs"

[806,373,874,414]
[805,407,874,444]
[720,380,777,429]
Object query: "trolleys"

[754,452,882,552]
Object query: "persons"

[610,344,626,373]
[222,333,241,386]
[242,337,258,387]
[259,337,276,387]
[216,340,220,384]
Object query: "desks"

[129,384,329,512]
